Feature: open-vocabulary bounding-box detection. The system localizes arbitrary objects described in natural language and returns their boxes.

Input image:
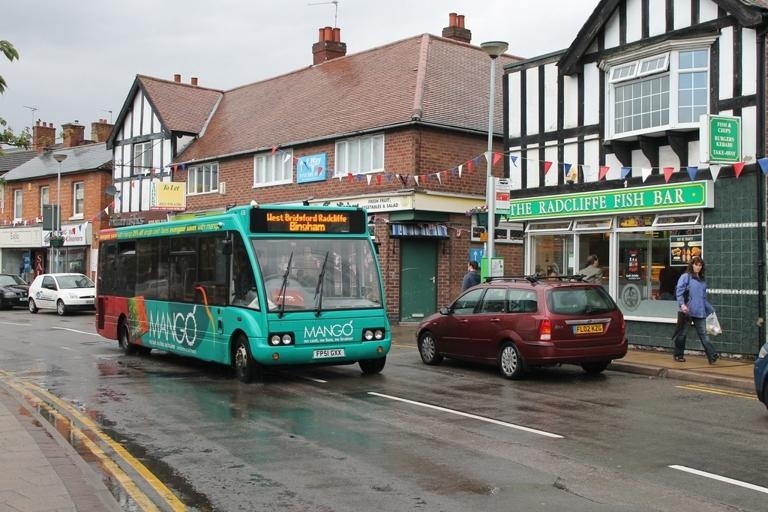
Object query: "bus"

[94,199,393,381]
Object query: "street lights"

[52,153,67,272]
[482,41,510,259]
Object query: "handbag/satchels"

[682,273,691,304]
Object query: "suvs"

[416,272,628,379]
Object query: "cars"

[0,272,30,307]
[26,274,96,314]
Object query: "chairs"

[555,293,578,313]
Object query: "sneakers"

[673,355,686,362]
[709,353,720,364]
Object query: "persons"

[656,255,681,301]
[542,269,559,281]
[461,260,481,295]
[671,255,722,366]
[230,247,268,307]
[577,254,604,306]
